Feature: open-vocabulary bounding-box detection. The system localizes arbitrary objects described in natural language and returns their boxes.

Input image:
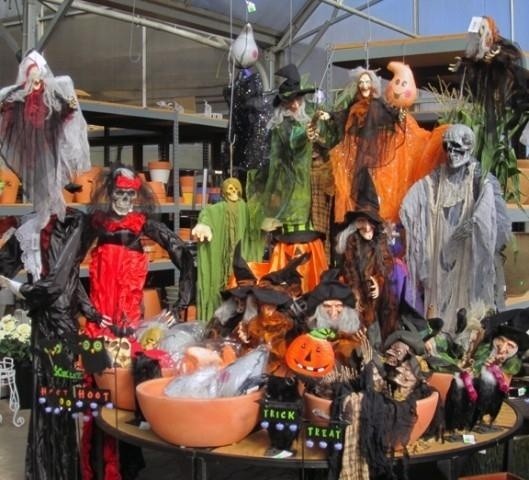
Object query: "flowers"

[0,315,34,363]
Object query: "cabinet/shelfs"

[324,23,529,321]
[0,0,277,322]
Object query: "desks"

[91,383,525,480]
